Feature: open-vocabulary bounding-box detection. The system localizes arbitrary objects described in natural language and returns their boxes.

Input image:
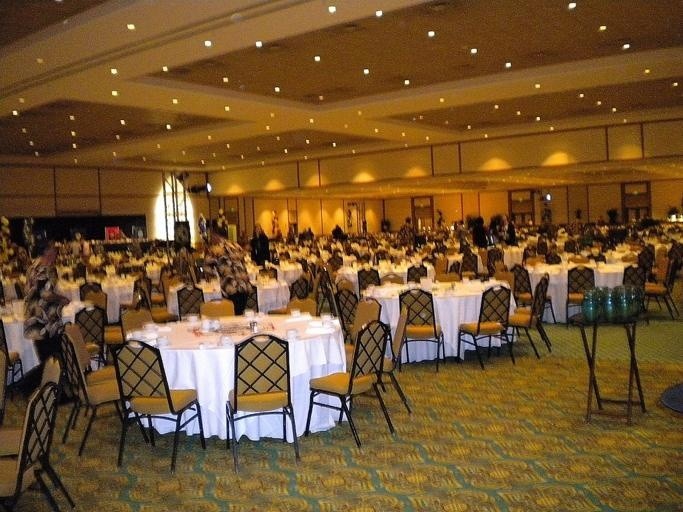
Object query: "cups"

[364,275,503,297]
[128,307,339,350]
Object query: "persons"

[469,213,519,250]
[22,238,73,408]
[201,224,254,316]
[396,214,419,251]
[248,223,272,267]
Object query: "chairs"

[225,332,303,467]
[0,353,66,489]
[112,339,206,473]
[57,322,149,461]
[0,383,78,511]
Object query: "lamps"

[189,181,213,194]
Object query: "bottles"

[580,286,642,321]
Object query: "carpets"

[0,346,683,511]
